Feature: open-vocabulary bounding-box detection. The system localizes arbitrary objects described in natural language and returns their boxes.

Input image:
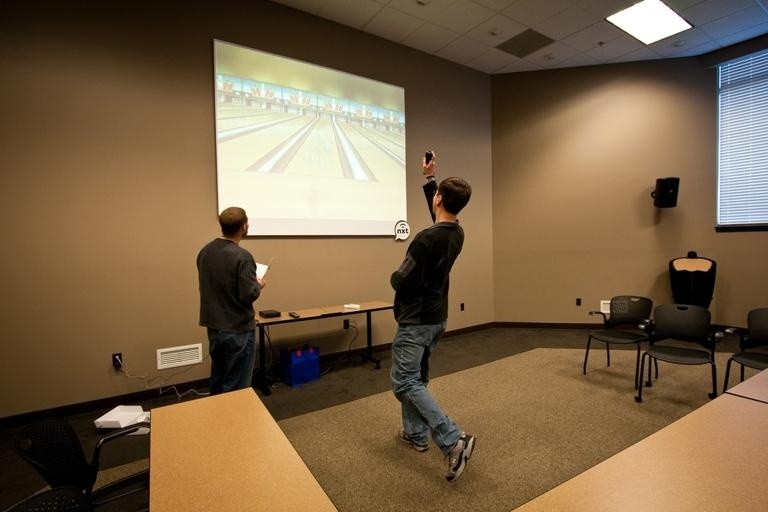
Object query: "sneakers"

[398,429,430,452]
[446,432,476,482]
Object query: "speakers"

[653,176,680,207]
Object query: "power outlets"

[112,352,122,369]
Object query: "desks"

[255,300,394,396]
[724,367,768,403]
[509,392,768,512]
[147,386,338,512]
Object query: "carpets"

[276,344,766,512]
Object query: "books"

[254,263,268,278]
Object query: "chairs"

[0,409,150,512]
[583,294,768,402]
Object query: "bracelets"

[425,176,434,180]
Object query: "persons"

[194,206,269,396]
[389,151,477,484]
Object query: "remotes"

[288,311,300,318]
